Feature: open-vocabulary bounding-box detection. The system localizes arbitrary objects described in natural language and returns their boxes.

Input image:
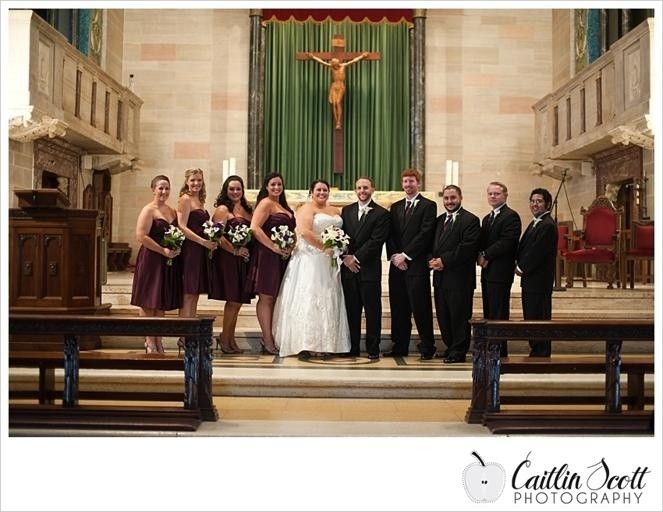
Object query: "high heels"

[215,335,236,355]
[144,340,159,355]
[260,336,281,356]
[176,339,186,357]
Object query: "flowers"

[162,225,186,265]
[321,225,350,266]
[228,224,254,262]
[271,225,295,260]
[201,216,226,260]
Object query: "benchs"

[501,357,654,411]
[9,351,184,405]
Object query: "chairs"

[562,196,624,289]
[623,220,654,289]
[557,221,581,286]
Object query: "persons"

[273,178,353,358]
[175,169,216,354]
[310,51,370,130]
[339,178,387,359]
[131,176,181,354]
[516,189,558,357]
[210,177,255,352]
[251,171,296,353]
[480,182,521,357]
[383,171,438,360]
[426,184,480,363]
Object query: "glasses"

[529,198,544,204]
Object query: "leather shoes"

[435,351,449,359]
[420,353,433,360]
[442,356,466,363]
[341,351,361,358]
[368,352,379,359]
[383,351,409,357]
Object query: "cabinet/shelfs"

[9,190,112,314]
